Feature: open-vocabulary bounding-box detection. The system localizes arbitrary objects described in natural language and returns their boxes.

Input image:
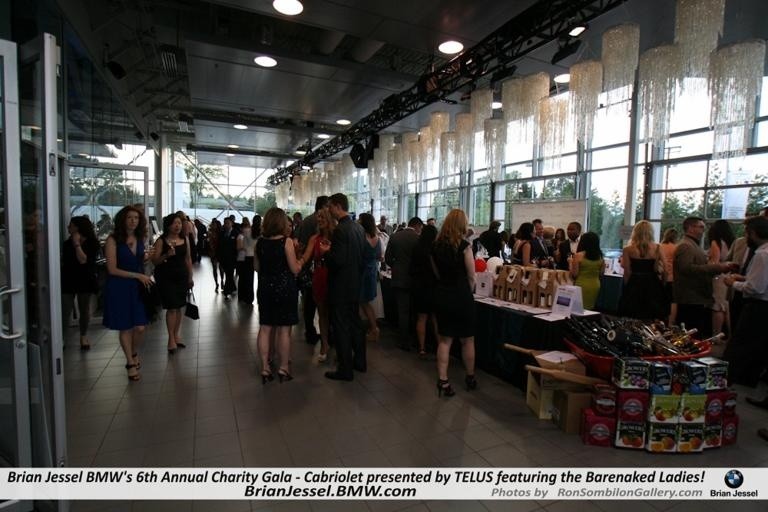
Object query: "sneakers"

[418,348,427,358]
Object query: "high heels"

[261,369,273,384]
[437,377,455,397]
[465,374,477,392]
[279,368,292,383]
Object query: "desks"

[596,273,623,311]
[379,272,601,392]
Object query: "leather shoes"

[306,326,380,381]
[746,395,768,408]
[81,338,89,349]
[168,342,186,354]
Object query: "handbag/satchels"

[297,267,313,289]
[185,301,199,319]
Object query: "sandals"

[126,352,141,381]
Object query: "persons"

[0,194,768,439]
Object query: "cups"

[319,240,331,247]
[167,239,176,248]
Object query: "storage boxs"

[526,349,739,454]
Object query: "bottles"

[673,322,725,355]
[493,285,552,307]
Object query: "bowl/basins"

[563,338,711,381]
